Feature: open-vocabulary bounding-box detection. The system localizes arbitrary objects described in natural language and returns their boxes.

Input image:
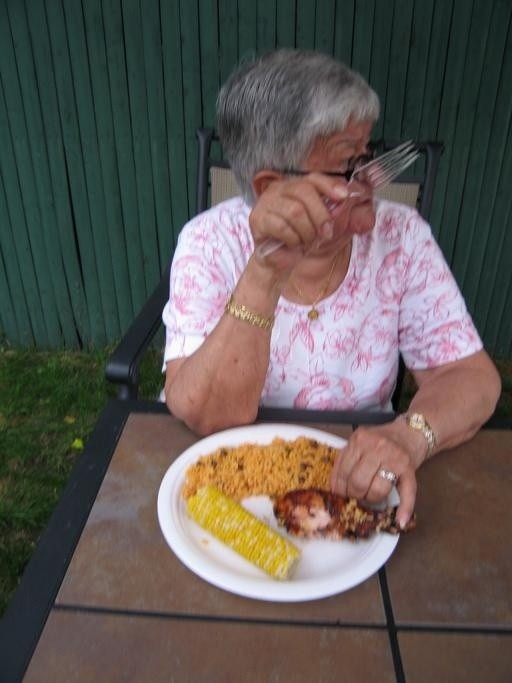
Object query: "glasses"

[272,138,390,198]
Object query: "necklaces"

[287,255,340,322]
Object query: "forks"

[253,140,421,257]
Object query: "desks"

[0,395,512,683]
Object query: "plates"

[155,421,401,604]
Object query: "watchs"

[393,403,435,464]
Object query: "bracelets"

[224,292,276,332]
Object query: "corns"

[187,487,302,587]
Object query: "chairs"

[104,125,442,414]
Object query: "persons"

[155,45,502,530]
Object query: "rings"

[374,468,399,485]
